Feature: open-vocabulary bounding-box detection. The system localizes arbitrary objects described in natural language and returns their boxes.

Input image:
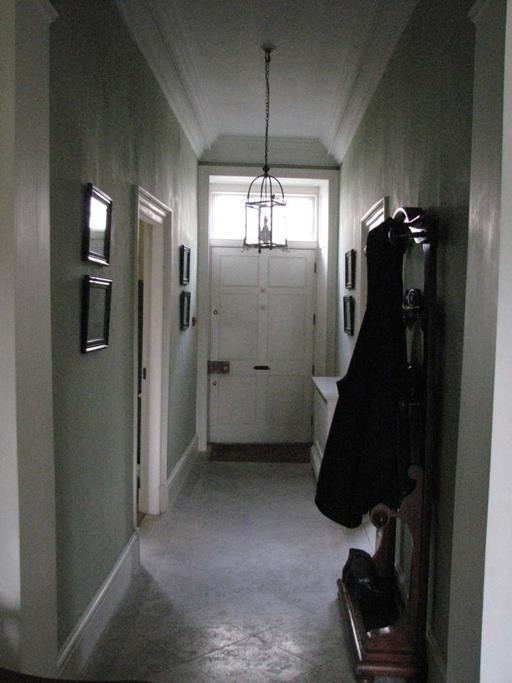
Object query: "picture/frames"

[81,182,113,353]
[341,249,354,335]
[179,244,191,330]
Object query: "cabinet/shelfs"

[308,375,342,483]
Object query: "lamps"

[242,44,287,252]
